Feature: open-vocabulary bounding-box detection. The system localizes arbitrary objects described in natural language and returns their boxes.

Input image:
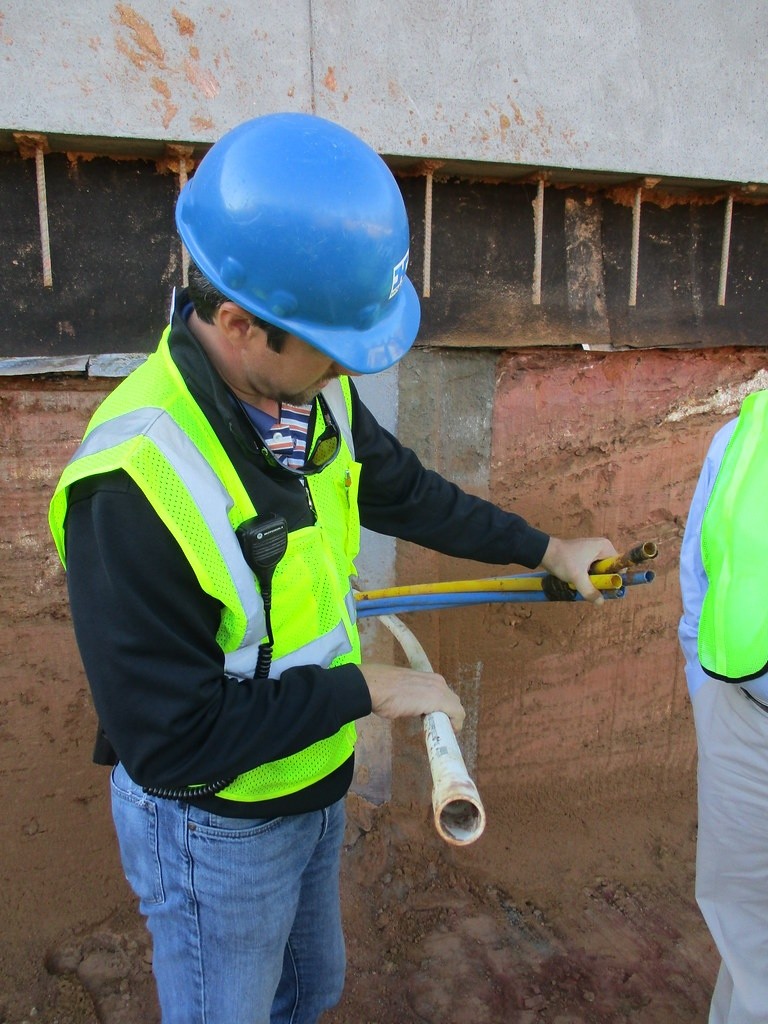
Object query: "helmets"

[174,112,421,373]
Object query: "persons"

[48,112,629,1024]
[676,389,768,1024]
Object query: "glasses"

[226,387,342,476]
